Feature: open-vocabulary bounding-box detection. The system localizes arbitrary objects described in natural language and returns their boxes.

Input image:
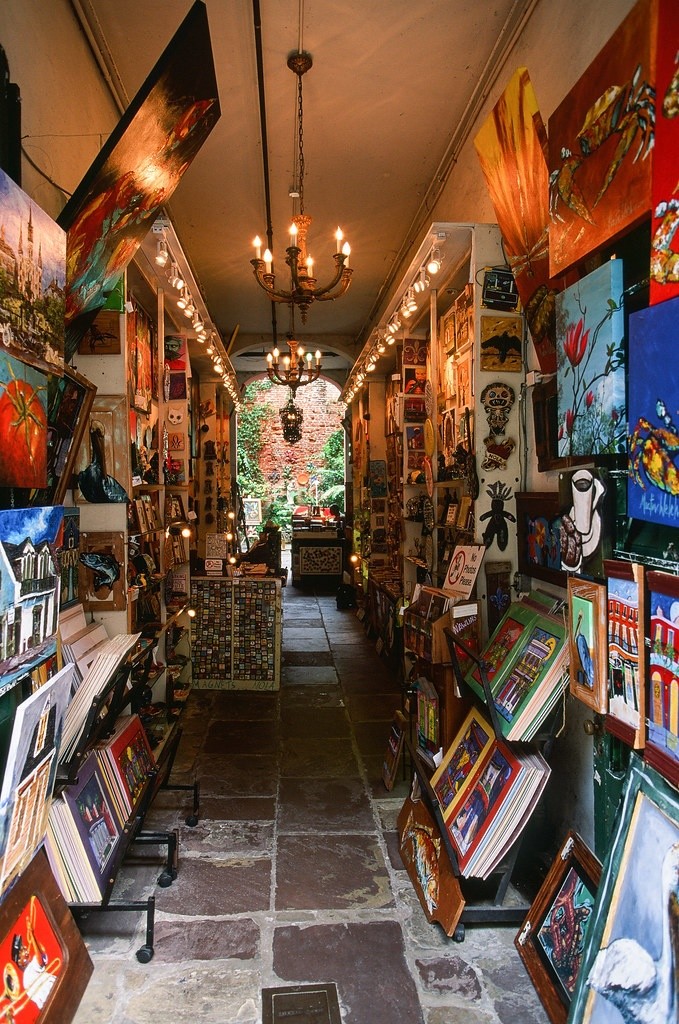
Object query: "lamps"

[177,284,189,308]
[168,261,184,289]
[401,298,411,318]
[155,241,168,266]
[389,314,402,334]
[352,376,363,387]
[376,339,386,353]
[184,296,195,317]
[358,366,366,380]
[380,331,395,345]
[279,334,303,445]
[210,354,222,363]
[406,288,418,312]
[364,361,375,372]
[192,312,204,332]
[197,329,206,343]
[251,54,354,325]
[414,267,430,293]
[267,277,322,398]
[372,348,380,361]
[427,249,442,274]
[207,340,215,354]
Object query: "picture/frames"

[0,0,679,1024]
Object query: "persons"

[12,921,57,1011]
[329,504,341,521]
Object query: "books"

[422,587,575,883]
[1,606,155,905]
